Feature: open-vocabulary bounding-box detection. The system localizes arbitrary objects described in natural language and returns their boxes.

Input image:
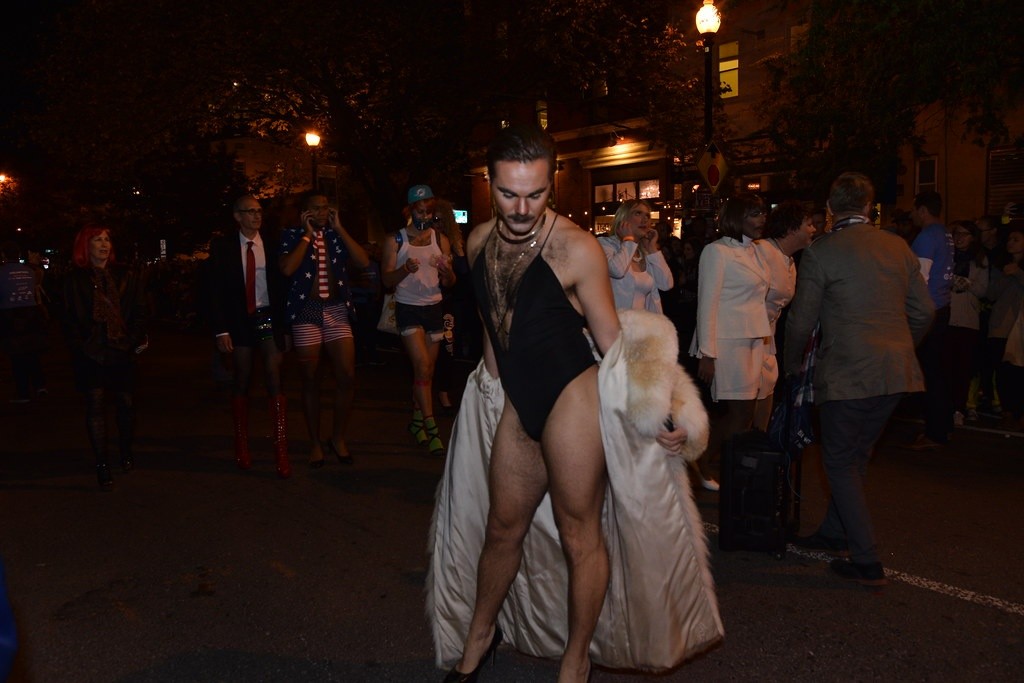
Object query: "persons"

[278,190,371,468]
[0,238,50,405]
[380,182,464,459]
[203,194,292,480]
[57,223,151,489]
[597,171,1024,590]
[423,123,721,683]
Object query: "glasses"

[979,227,992,232]
[954,231,971,237]
[238,208,264,215]
[745,209,767,218]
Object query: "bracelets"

[404,264,410,273]
[302,237,310,243]
[622,236,634,241]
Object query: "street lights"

[306,126,326,200]
[694,0,723,263]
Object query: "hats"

[408,184,434,205]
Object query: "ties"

[244,240,256,317]
[313,227,330,299]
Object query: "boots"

[234,397,253,467]
[270,397,291,475]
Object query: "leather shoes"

[799,532,851,550]
[830,558,888,587]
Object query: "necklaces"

[494,221,545,320]
[495,213,546,244]
[632,251,643,263]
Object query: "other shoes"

[436,399,456,414]
[967,407,979,426]
[900,433,945,449]
[121,447,135,472]
[984,407,1004,418]
[94,460,114,487]
[953,410,965,427]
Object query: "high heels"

[327,438,353,467]
[688,458,721,491]
[442,623,503,683]
[407,418,428,446]
[428,425,447,463]
[308,458,325,467]
